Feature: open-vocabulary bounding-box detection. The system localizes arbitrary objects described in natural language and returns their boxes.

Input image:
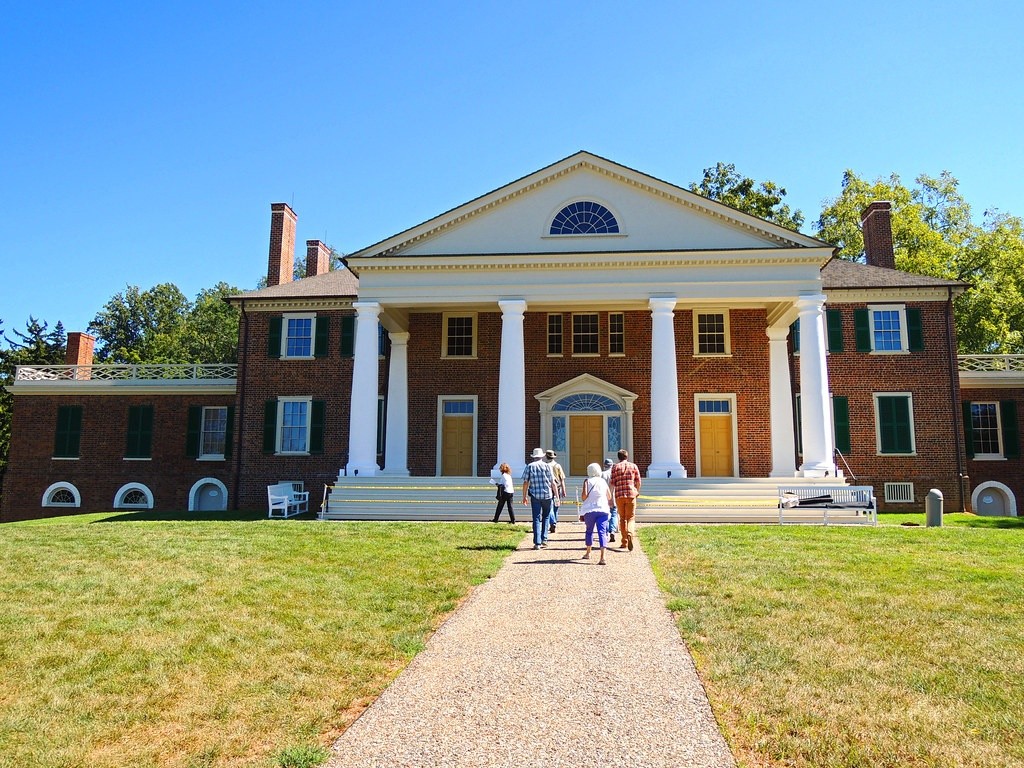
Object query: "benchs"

[777,485,877,528]
[267,482,309,518]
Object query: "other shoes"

[508,521,515,524]
[598,559,606,564]
[627,532,634,550]
[582,554,589,559]
[488,519,498,523]
[609,532,615,541]
[549,524,556,533]
[620,544,626,548]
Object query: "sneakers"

[533,544,540,549]
[541,541,548,547]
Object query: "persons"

[600,458,618,542]
[491,463,516,524]
[543,449,567,533]
[610,449,642,551]
[580,463,612,566]
[521,448,560,550]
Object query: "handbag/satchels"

[580,515,585,522]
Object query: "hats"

[545,449,557,457]
[603,459,613,467]
[530,447,546,458]
